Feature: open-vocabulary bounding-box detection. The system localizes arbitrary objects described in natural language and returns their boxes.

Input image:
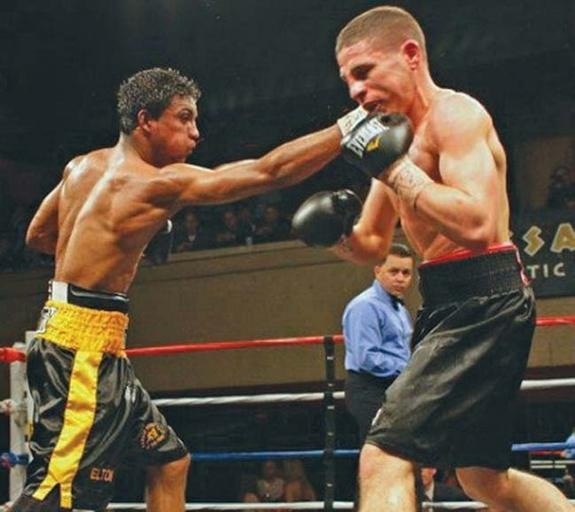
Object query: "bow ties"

[387,291,405,311]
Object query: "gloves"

[339,112,413,178]
[289,188,363,247]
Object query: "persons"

[0,67,381,511]
[341,244,424,512]
[0,59,575,279]
[289,7,575,512]
[240,430,574,512]
